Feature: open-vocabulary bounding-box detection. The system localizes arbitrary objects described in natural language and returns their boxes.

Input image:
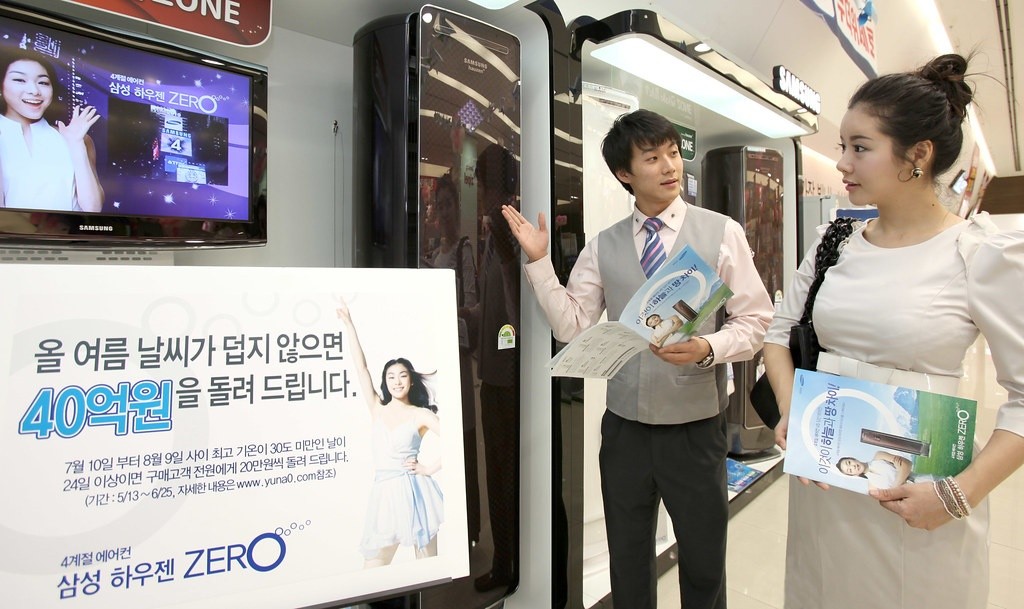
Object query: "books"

[781,368,986,494]
[549,244,736,379]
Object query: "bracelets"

[933,474,973,520]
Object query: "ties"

[639,216,667,280]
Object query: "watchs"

[695,345,715,367]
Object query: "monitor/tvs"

[0,0,268,252]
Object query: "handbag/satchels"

[749,322,819,431]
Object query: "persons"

[835,450,948,494]
[418,146,571,609]
[645,313,692,353]
[337,297,456,568]
[501,109,787,609]
[130,196,267,237]
[0,47,106,213]
[763,53,1024,609]
